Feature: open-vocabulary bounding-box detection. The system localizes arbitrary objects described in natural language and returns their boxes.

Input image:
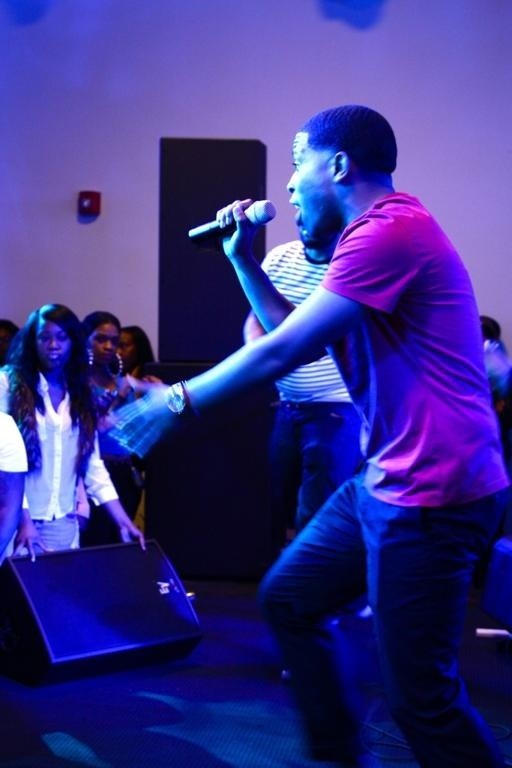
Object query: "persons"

[110,102,512,766]
[457,313,512,718]
[243,239,368,682]
[0,298,166,681]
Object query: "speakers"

[0,537,205,684]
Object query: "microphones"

[188,200,277,240]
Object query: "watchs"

[165,380,195,422]
